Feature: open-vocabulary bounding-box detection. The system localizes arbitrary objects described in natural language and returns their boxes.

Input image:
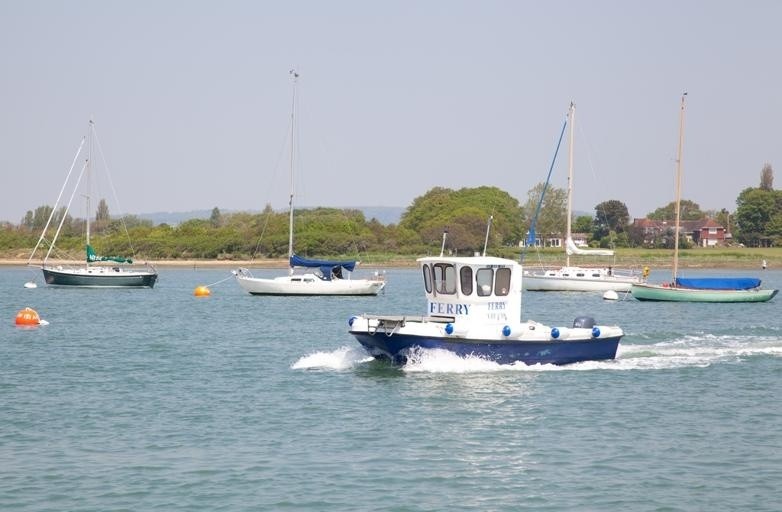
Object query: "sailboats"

[515,101,645,292]
[231,64,389,299]
[25,112,160,290]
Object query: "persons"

[453,269,483,296]
[761,258,767,271]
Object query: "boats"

[628,95,778,303]
[349,211,624,365]
